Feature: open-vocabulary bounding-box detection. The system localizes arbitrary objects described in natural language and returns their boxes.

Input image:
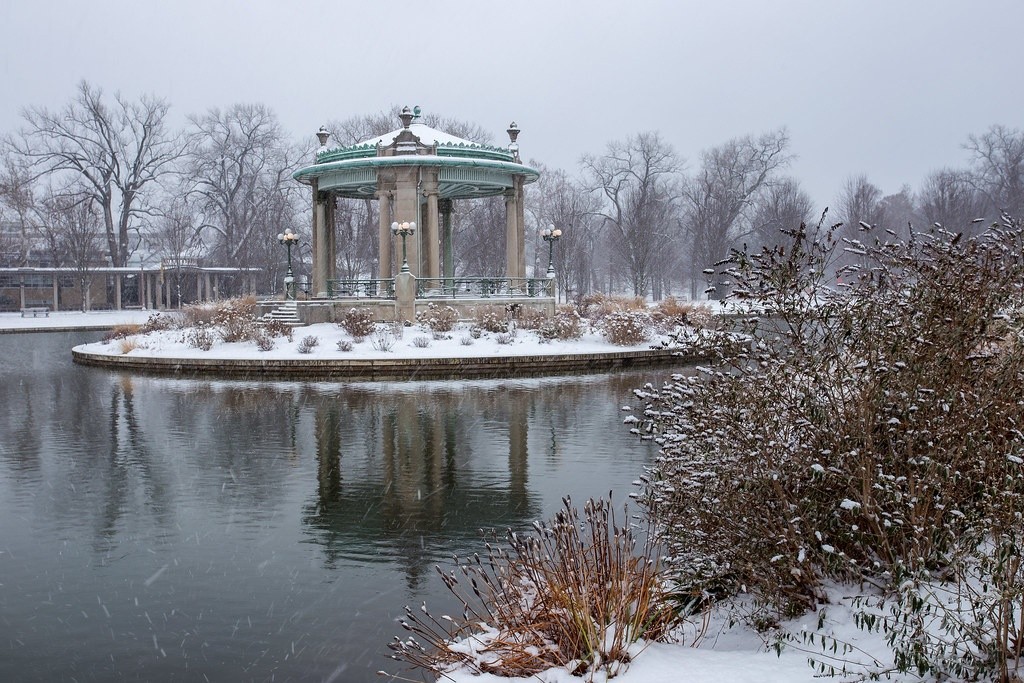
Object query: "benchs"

[20,307,50,318]
